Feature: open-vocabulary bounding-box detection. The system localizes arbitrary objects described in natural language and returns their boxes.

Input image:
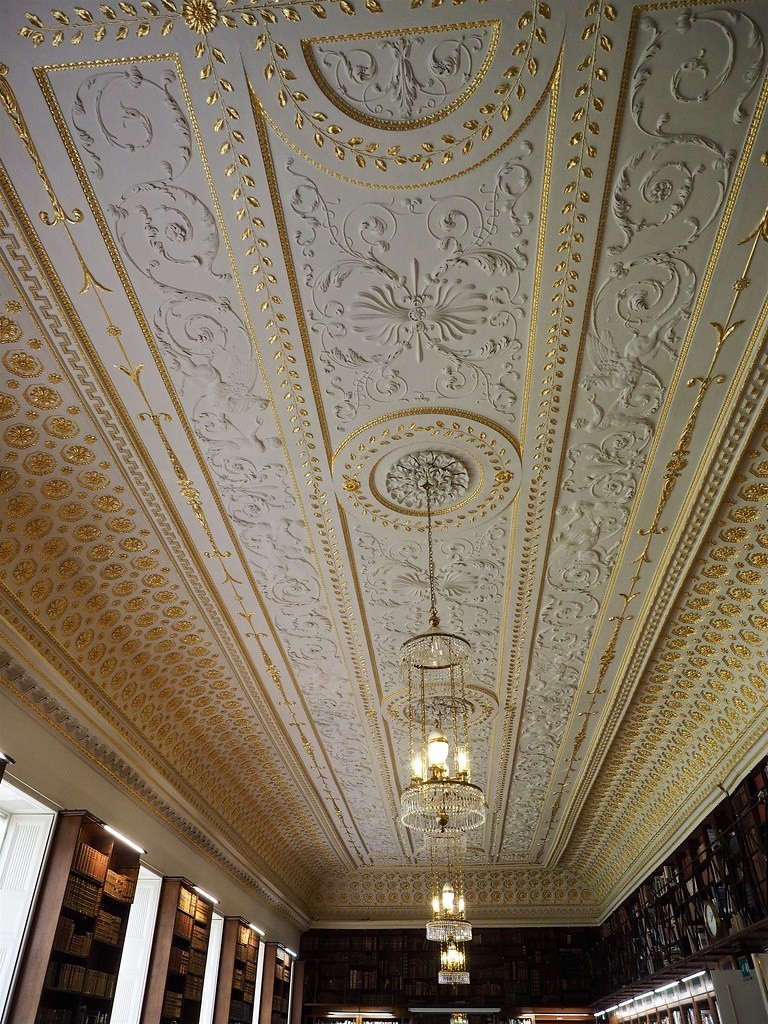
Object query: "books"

[301,758,767,1024]
[35,842,135,1024]
[229,923,258,1022]
[272,948,292,1024]
[161,885,211,1020]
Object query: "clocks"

[702,899,722,939]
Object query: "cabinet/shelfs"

[294,959,317,1024]
[6,809,141,1024]
[137,876,213,1024]
[259,942,292,1024]
[298,925,600,1012]
[600,752,768,997]
[212,915,260,1024]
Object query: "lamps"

[395,475,487,998]
[282,947,297,958]
[246,922,268,937]
[407,1007,502,1013]
[654,980,681,993]
[95,821,147,856]
[593,1010,605,1017]
[617,998,635,1007]
[634,991,654,1001]
[604,1004,619,1013]
[681,969,707,983]
[191,885,221,904]
